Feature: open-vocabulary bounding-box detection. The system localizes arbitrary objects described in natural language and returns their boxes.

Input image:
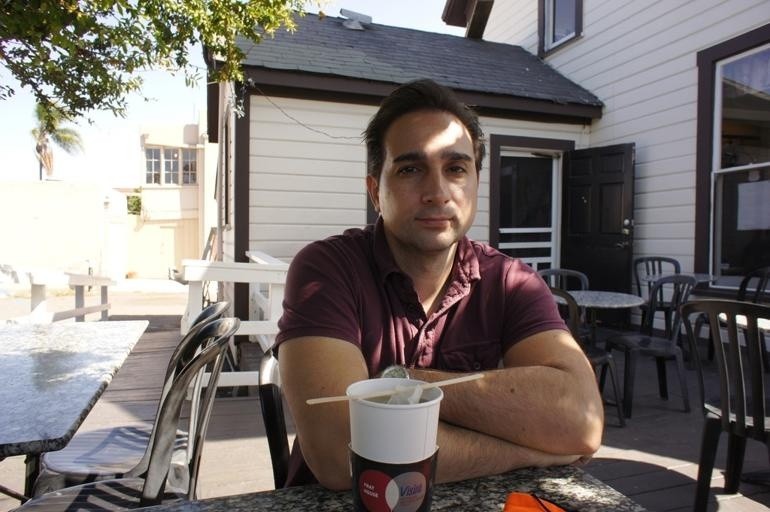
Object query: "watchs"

[377,366,412,379]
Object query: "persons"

[271,79,604,492]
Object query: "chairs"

[40,302,238,484]
[670,298,769,512]
[539,268,588,291]
[258,346,290,490]
[13,316,242,508]
[633,257,685,360]
[599,275,696,416]
[737,268,769,303]
[547,287,625,427]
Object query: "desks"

[102,459,646,512]
[537,290,645,353]
[0,320,150,502]
